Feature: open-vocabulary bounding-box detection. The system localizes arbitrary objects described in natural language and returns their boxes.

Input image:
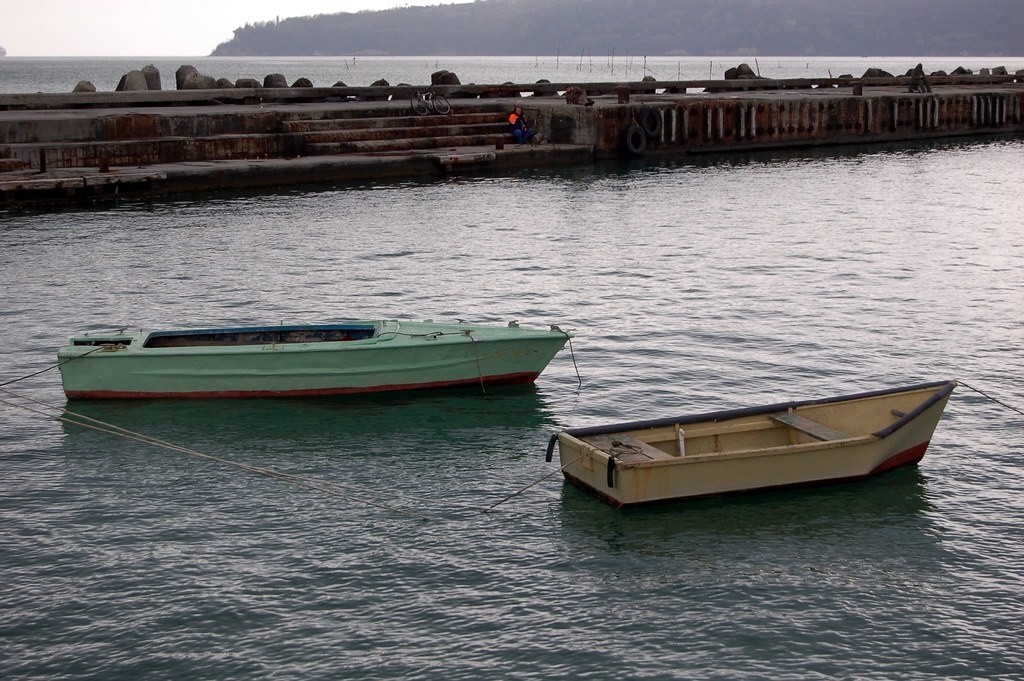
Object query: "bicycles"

[410,83,450,115]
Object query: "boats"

[56,314,575,405]
[545,379,962,512]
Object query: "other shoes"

[527,141,530,145]
[519,141,522,145]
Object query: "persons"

[510,105,534,144]
[909,62,932,93]
[565,84,595,106]
